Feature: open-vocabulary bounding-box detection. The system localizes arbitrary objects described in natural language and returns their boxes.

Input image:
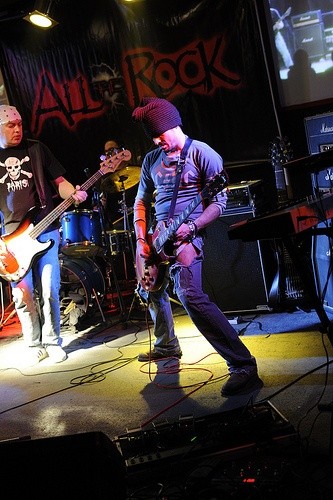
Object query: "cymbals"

[111,165,141,192]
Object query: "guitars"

[0,146,132,282]
[135,170,229,294]
[268,137,313,313]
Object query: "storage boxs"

[226,180,265,211]
[202,209,281,313]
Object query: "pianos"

[228,145,333,347]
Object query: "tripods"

[87,182,185,338]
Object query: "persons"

[134,98,265,398]
[0,105,88,363]
[94,141,139,309]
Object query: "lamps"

[22,0,59,32]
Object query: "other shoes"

[45,345,67,363]
[29,347,47,364]
[221,367,258,395]
[138,349,183,362]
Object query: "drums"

[101,229,131,256]
[59,209,101,257]
[58,252,114,325]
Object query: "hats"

[131,97,182,139]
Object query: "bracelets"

[135,238,146,243]
[188,221,197,234]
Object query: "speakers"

[199,180,276,313]
[0,432,133,500]
